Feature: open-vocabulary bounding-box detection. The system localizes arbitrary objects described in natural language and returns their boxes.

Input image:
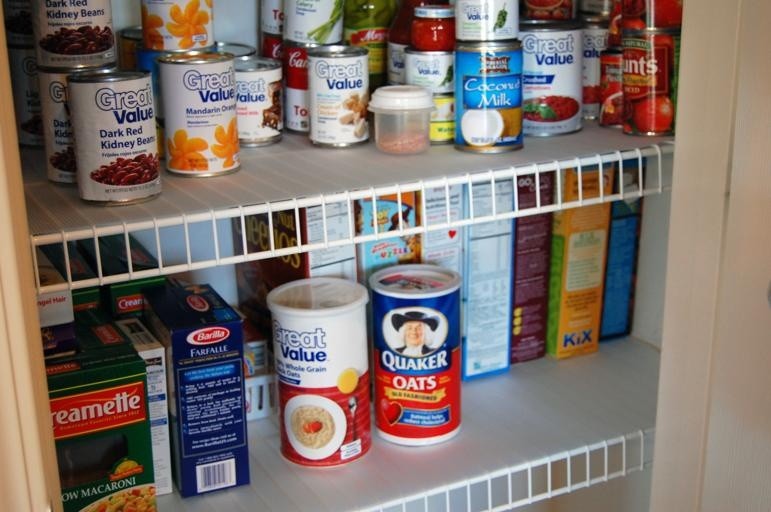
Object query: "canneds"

[9,1,683,207]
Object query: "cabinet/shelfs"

[1,0,674,512]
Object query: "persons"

[389,310,441,356]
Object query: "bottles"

[367,82,439,158]
